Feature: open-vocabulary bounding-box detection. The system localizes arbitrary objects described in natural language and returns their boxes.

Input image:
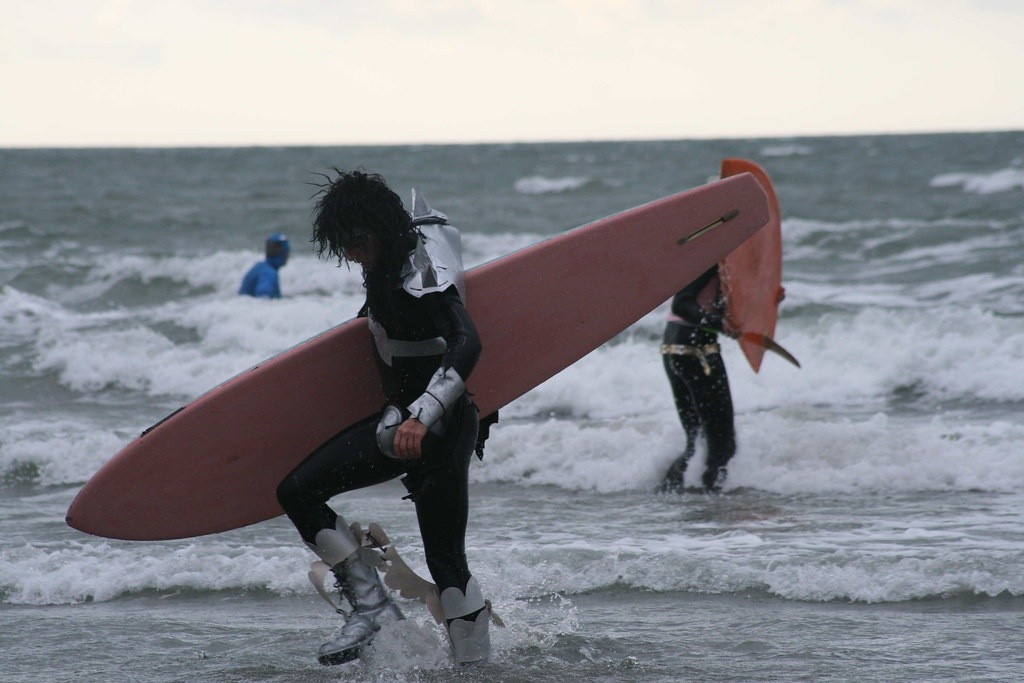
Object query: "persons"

[236,234,292,298]
[275,167,498,668]
[652,262,785,494]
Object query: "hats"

[266,235,290,253]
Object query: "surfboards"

[65,172,771,545]
[719,157,781,376]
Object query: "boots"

[317,547,406,666]
[442,608,491,667]
